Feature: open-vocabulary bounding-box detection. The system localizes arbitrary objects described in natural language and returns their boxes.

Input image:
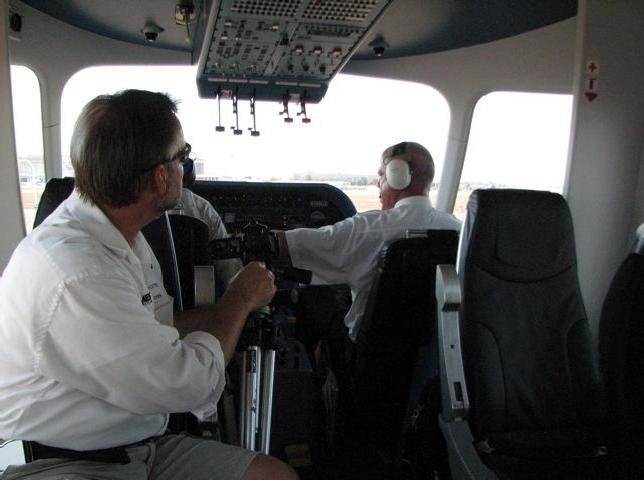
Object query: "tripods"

[237,316,277,454]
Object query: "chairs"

[141,215,209,316]
[600,221,644,480]
[323,230,459,478]
[436,190,618,480]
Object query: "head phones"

[384,141,412,192]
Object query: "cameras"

[211,224,281,266]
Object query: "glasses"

[147,143,191,173]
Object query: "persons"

[155,152,244,290]
[1,89,301,479]
[269,141,463,379]
